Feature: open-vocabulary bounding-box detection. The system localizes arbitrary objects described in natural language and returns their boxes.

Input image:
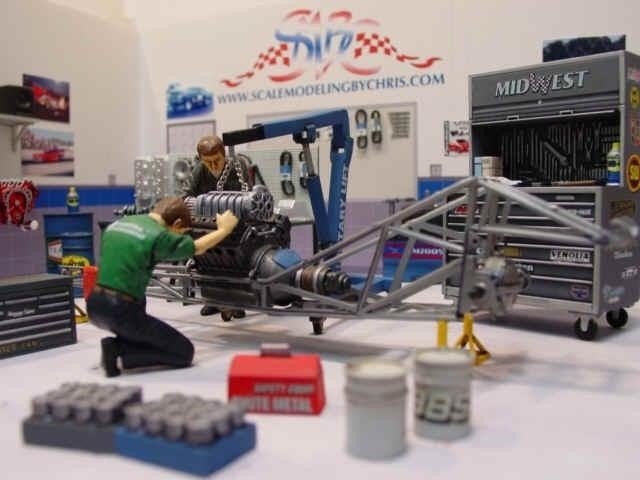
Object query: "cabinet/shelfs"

[446,50,638,338]
